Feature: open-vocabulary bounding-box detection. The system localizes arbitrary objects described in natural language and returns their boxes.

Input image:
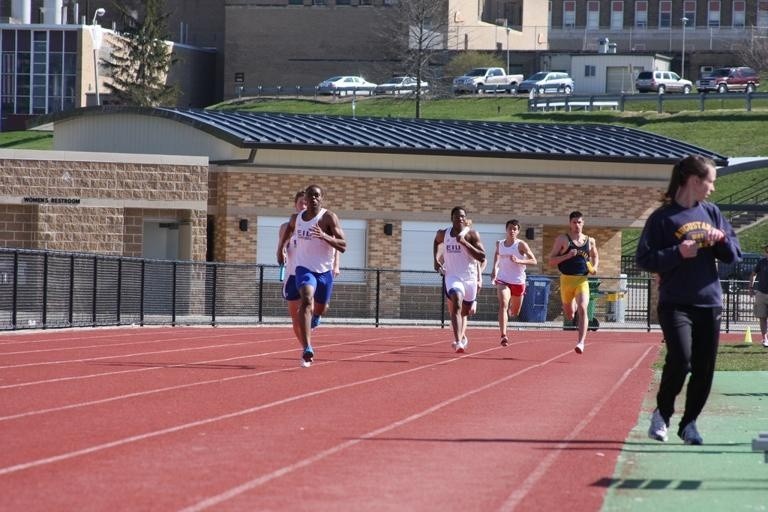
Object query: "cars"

[315,74,378,97]
[375,76,428,98]
[515,72,574,96]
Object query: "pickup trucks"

[451,66,524,97]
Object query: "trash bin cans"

[599,289,627,324]
[562,278,600,332]
[518,274,552,323]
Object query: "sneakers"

[575,343,584,353]
[500,336,508,346]
[678,421,703,444]
[300,345,314,368]
[649,408,668,442]
[451,336,467,352]
[312,315,321,328]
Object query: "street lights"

[679,16,689,78]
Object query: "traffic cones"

[744,327,752,343]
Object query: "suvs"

[696,66,760,94]
[634,71,692,96]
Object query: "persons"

[276,184,346,363]
[748,244,768,348]
[278,190,340,369]
[437,218,487,347]
[547,211,599,354]
[433,207,486,353]
[634,154,744,445]
[490,218,538,347]
